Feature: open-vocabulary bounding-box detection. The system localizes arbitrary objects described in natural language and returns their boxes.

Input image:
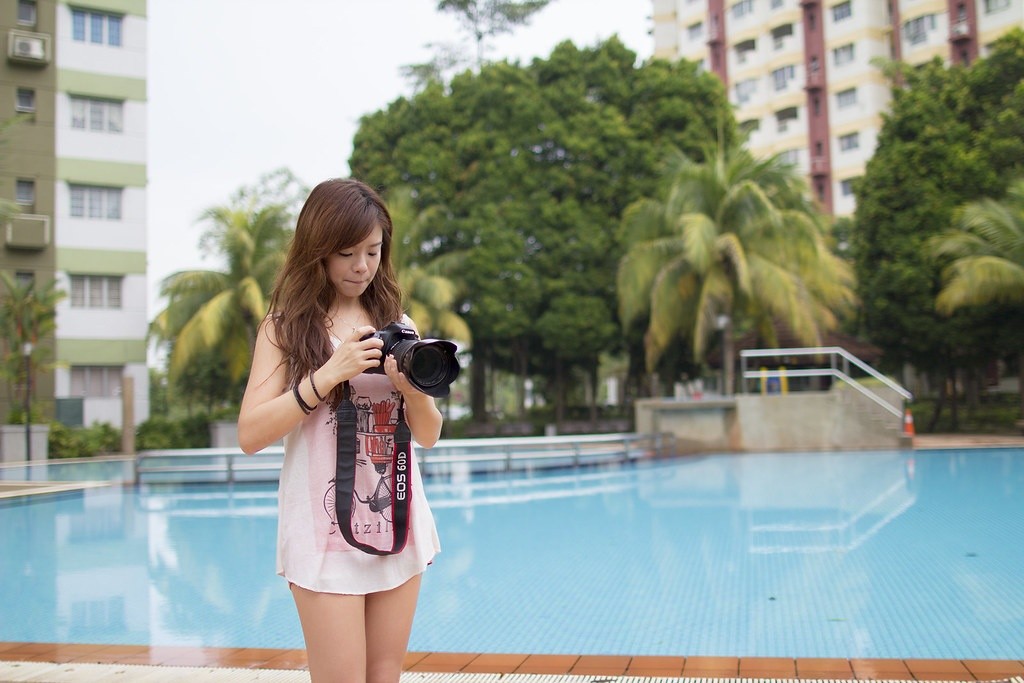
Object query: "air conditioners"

[13,35,44,59]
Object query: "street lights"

[717,311,729,403]
[25,342,34,480]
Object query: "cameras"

[358,321,460,398]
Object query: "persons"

[238,179,443,683]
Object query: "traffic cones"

[904,408,920,444]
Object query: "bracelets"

[310,372,326,402]
[293,387,310,415]
[295,386,317,411]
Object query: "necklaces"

[333,306,363,330]
